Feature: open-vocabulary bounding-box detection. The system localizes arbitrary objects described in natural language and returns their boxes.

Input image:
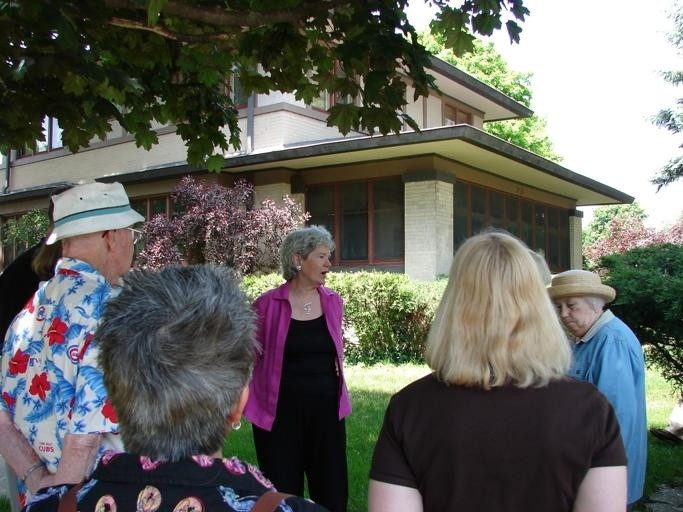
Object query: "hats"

[45,181,145,245]
[547,268,616,303]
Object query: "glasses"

[102,225,146,242]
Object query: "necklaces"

[291,287,319,316]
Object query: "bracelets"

[22,461,45,485]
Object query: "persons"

[20,263,325,512]
[0,182,146,512]
[546,270,648,512]
[0,185,75,355]
[367,233,628,512]
[227,225,352,512]
